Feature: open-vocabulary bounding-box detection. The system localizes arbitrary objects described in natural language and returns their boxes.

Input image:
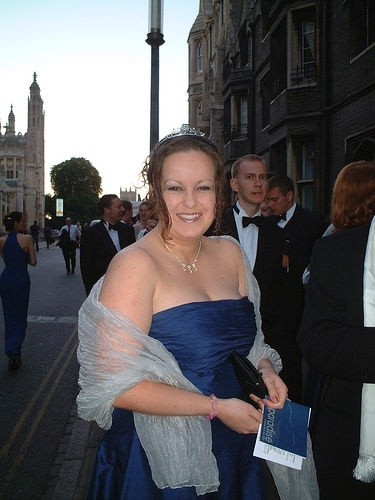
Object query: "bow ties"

[272,213,288,222]
[234,206,266,228]
[103,220,118,231]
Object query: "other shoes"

[8,358,21,369]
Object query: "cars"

[50,229,60,239]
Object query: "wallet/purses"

[231,351,269,408]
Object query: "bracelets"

[208,394,217,419]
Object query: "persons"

[266,174,313,404]
[203,154,284,317]
[80,195,156,297]
[59,217,81,275]
[30,220,40,252]
[44,223,52,249]
[0,211,37,371]
[297,159,375,500]
[76,222,89,233]
[261,201,275,217]
[76,127,319,500]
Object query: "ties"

[68,226,70,236]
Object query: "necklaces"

[157,224,202,274]
